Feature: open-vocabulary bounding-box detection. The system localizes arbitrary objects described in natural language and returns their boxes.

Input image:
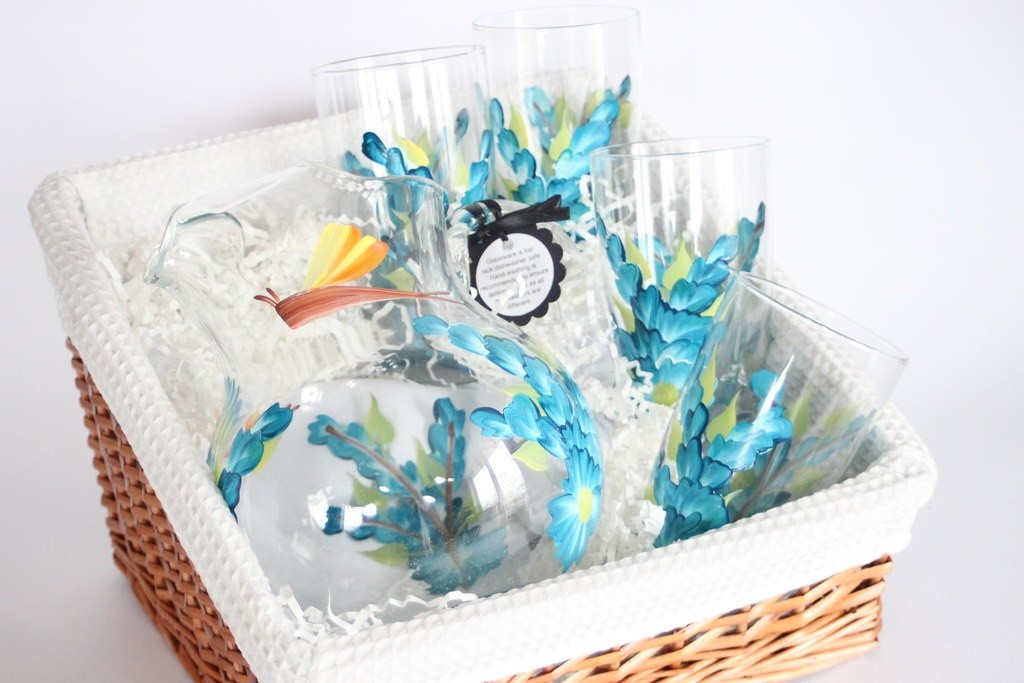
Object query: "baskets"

[26,112,941,683]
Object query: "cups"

[471,4,641,252]
[311,45,492,227]
[645,269,909,551]
[589,134,773,411]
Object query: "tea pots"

[144,159,606,635]
